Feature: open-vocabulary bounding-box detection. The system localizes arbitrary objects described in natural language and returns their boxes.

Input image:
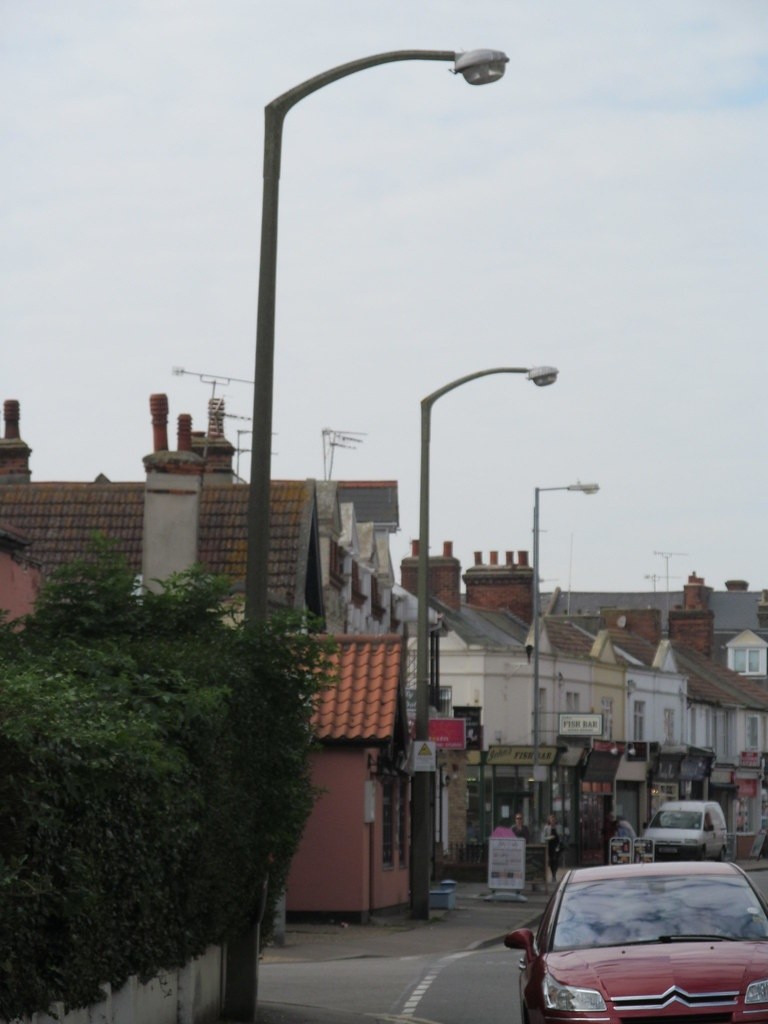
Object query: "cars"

[502,859,768,1024]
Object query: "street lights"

[215,45,507,1018]
[530,484,604,842]
[411,366,554,922]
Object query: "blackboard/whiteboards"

[489,836,527,890]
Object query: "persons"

[603,813,637,861]
[510,812,564,882]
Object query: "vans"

[657,801,727,861]
